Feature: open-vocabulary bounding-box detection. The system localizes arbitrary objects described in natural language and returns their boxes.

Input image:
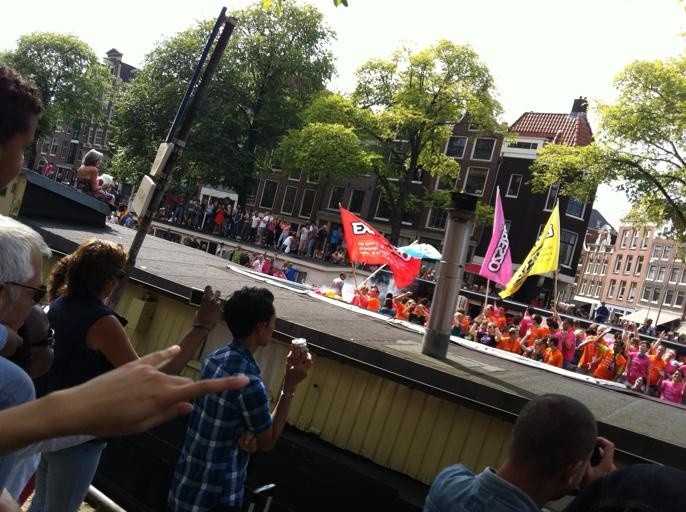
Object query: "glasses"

[110,267,129,285]
[2,278,48,304]
[30,326,57,350]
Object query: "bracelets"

[281,390,295,399]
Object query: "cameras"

[189,288,205,309]
[590,445,604,468]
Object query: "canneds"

[291,338,307,358]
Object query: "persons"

[423,393,617,512]
[108,187,138,229]
[169,284,312,512]
[451,301,685,404]
[351,285,431,328]
[419,266,436,281]
[0,64,44,356]
[29,241,221,512]
[0,346,249,453]
[164,192,346,282]
[77,150,114,204]
[42,156,76,187]
[1,216,98,512]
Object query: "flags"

[338,202,421,289]
[498,201,560,299]
[478,189,513,288]
[398,243,443,261]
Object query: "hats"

[83,149,104,163]
